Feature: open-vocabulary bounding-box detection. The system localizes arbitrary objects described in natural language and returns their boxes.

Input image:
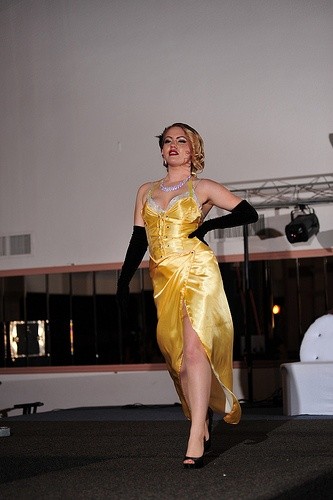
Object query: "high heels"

[182,436,205,470]
[204,408,213,451]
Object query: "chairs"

[280,314,333,417]
[0,401,44,417]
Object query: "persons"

[115,122,258,468]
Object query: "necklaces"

[156,174,193,192]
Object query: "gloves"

[188,200,258,246]
[116,225,149,311]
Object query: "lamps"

[284,206,319,244]
